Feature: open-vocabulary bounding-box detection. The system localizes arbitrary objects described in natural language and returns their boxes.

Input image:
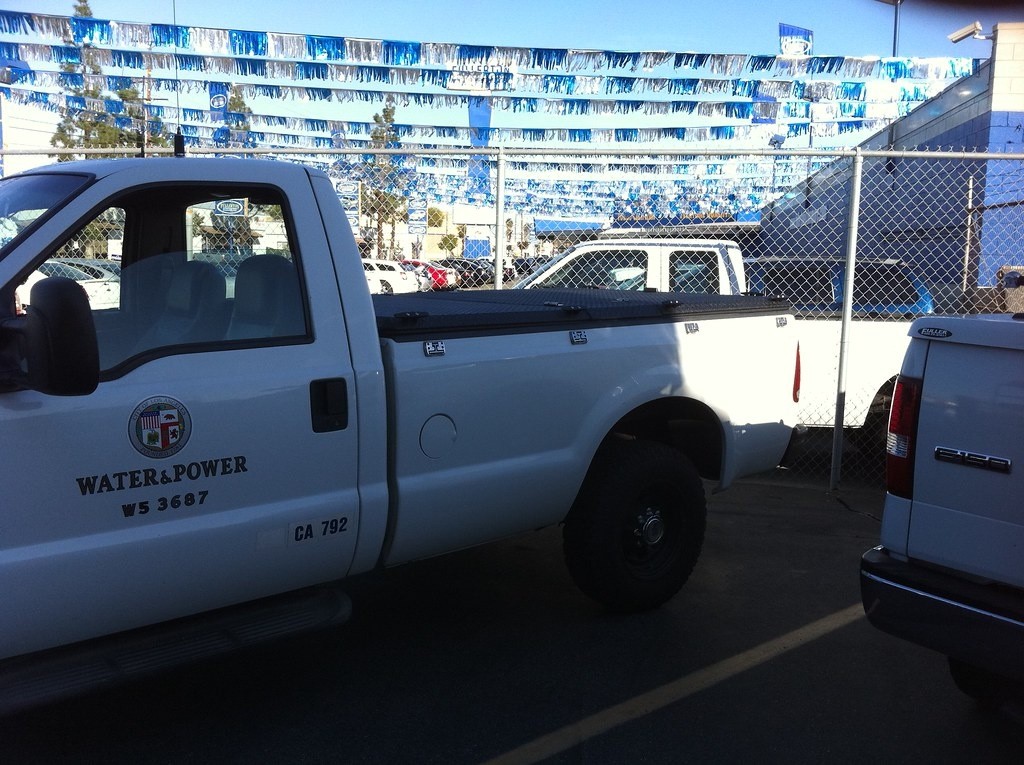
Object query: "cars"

[360,254,549,295]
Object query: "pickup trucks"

[511,237,752,298]
[0,152,812,658]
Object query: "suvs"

[613,257,936,316]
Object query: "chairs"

[221,255,308,341]
[123,260,227,360]
